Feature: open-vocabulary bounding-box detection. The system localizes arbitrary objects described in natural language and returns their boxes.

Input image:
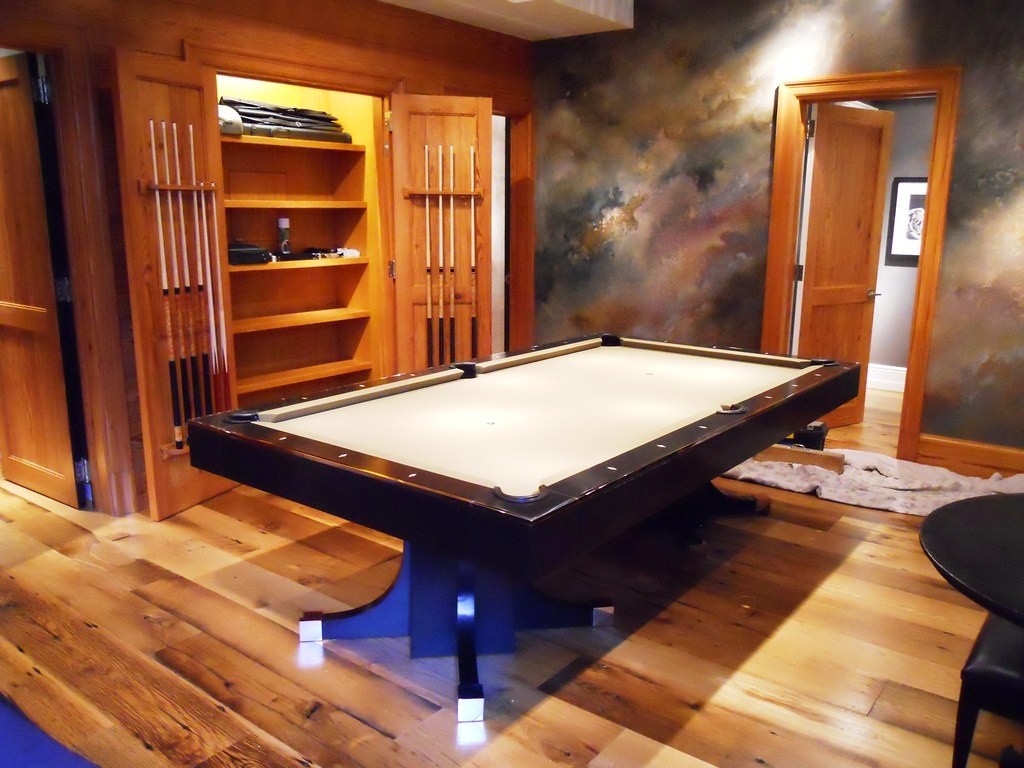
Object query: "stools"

[952,613,1024,768]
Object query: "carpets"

[728,448,1024,516]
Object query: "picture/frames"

[885,176,928,267]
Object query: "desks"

[186,334,863,721]
[918,492,1024,628]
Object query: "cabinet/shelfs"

[105,38,494,522]
